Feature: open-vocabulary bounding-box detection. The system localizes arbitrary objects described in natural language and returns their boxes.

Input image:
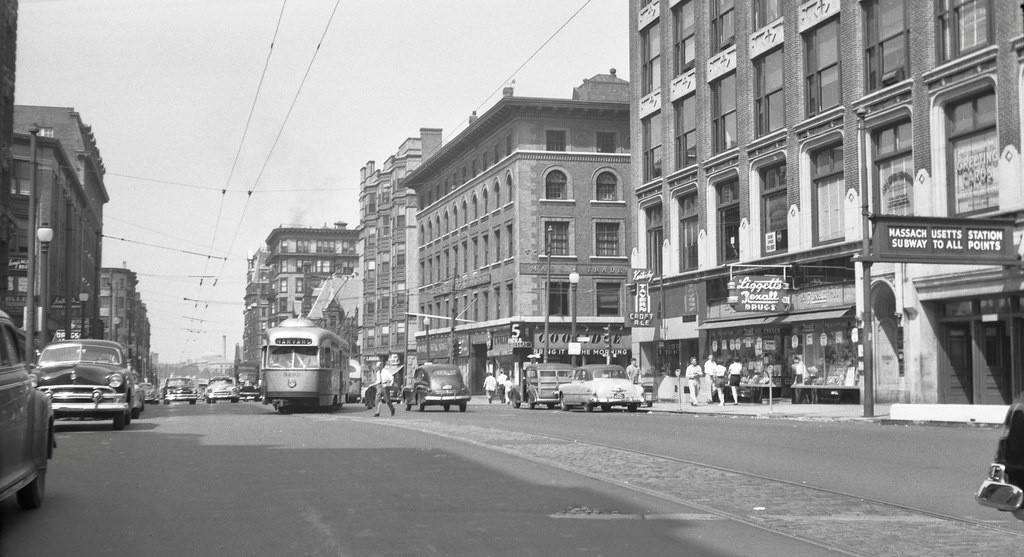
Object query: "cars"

[401,364,471,412]
[0,310,56,511]
[163,378,197,404]
[509,363,575,409]
[144,382,159,403]
[556,364,645,412]
[204,376,239,404]
[30,338,144,430]
[239,382,261,402]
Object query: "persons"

[625,358,641,385]
[504,377,513,405]
[368,361,395,417]
[496,371,507,403]
[686,357,704,407]
[727,356,743,405]
[714,359,727,406]
[704,354,718,404]
[484,373,496,405]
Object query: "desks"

[738,384,781,403]
[792,384,859,404]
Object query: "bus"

[261,316,350,413]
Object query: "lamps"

[619,326,624,331]
[536,324,540,331]
[581,323,590,332]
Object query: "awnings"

[695,305,851,330]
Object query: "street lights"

[356,340,361,365]
[569,269,580,366]
[423,316,431,364]
[113,316,120,342]
[79,288,89,338]
[37,220,53,347]
